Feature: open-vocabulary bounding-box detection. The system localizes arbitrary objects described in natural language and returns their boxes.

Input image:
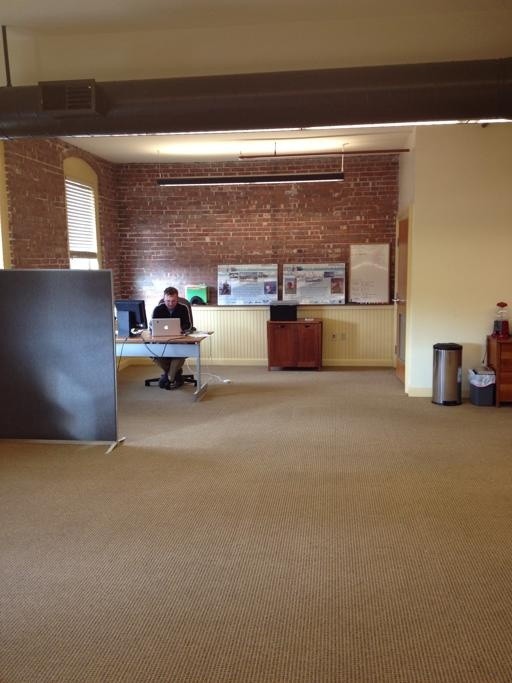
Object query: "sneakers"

[159,373,176,389]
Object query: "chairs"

[144,297,198,389]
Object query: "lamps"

[153,151,345,187]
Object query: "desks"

[114,331,215,401]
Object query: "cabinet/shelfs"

[486,334,512,407]
[265,318,323,370]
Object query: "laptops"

[151,318,182,337]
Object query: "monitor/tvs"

[113,298,148,337]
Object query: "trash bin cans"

[467,368,495,405]
[431,342,462,406]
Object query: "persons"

[151,287,192,389]
